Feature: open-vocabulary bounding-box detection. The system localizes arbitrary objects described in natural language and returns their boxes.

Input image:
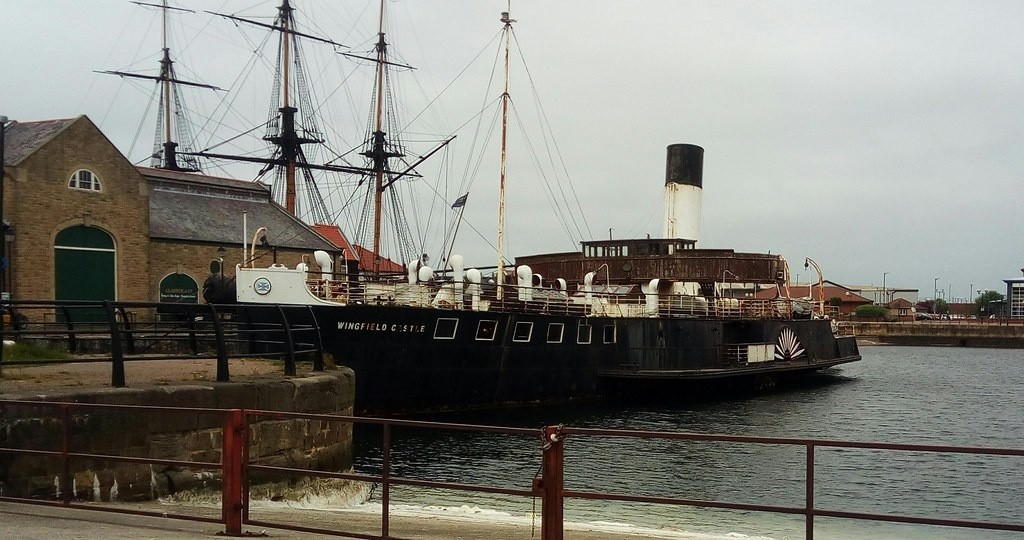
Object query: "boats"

[212,238,862,406]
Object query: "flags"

[451,194,467,209]
